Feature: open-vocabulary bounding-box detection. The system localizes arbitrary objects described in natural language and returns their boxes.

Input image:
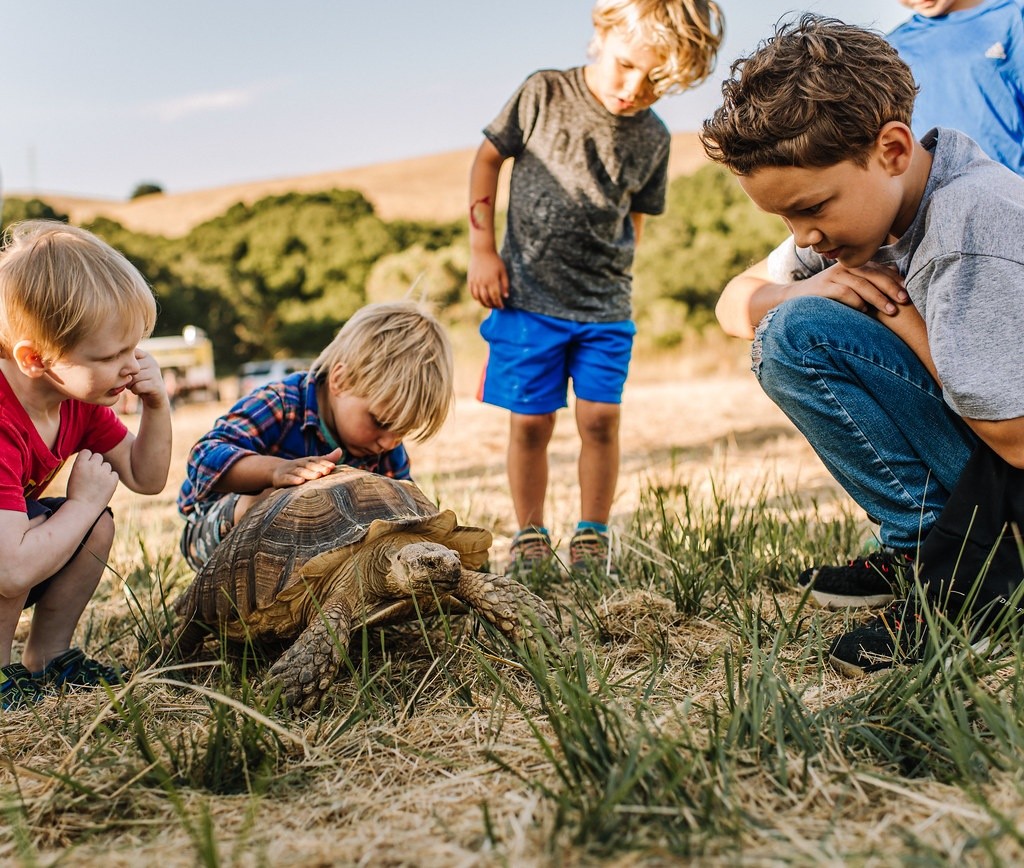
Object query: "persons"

[173,290,458,575]
[879,0,1024,179]
[1,217,175,711]
[464,0,726,579]
[697,9,1024,678]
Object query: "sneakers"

[30,648,132,693]
[503,533,553,582]
[0,662,44,708]
[571,533,610,585]
[831,591,1023,679]
[797,549,937,608]
